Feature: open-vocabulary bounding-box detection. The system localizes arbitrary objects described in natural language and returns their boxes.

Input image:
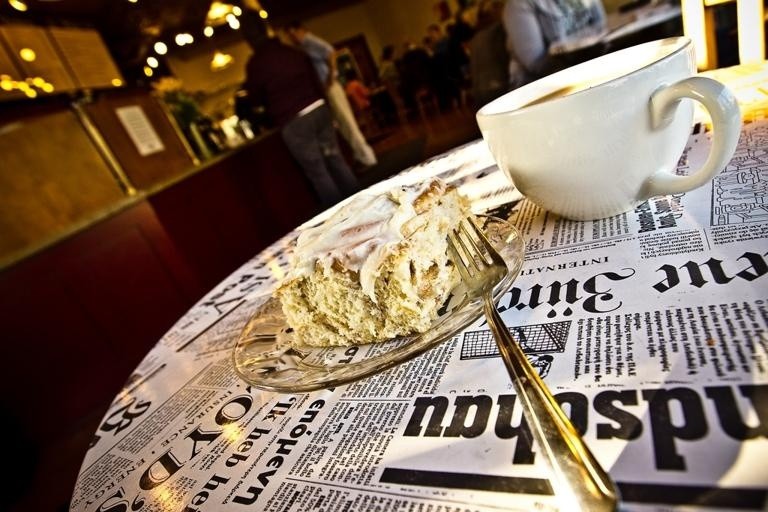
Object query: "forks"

[448,216,625,512]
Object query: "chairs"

[680,0,768,74]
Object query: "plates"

[232,212,527,392]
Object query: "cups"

[474,36,743,220]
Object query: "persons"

[240,9,361,206]
[286,15,377,173]
[344,0,608,118]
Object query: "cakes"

[275,175,481,348]
